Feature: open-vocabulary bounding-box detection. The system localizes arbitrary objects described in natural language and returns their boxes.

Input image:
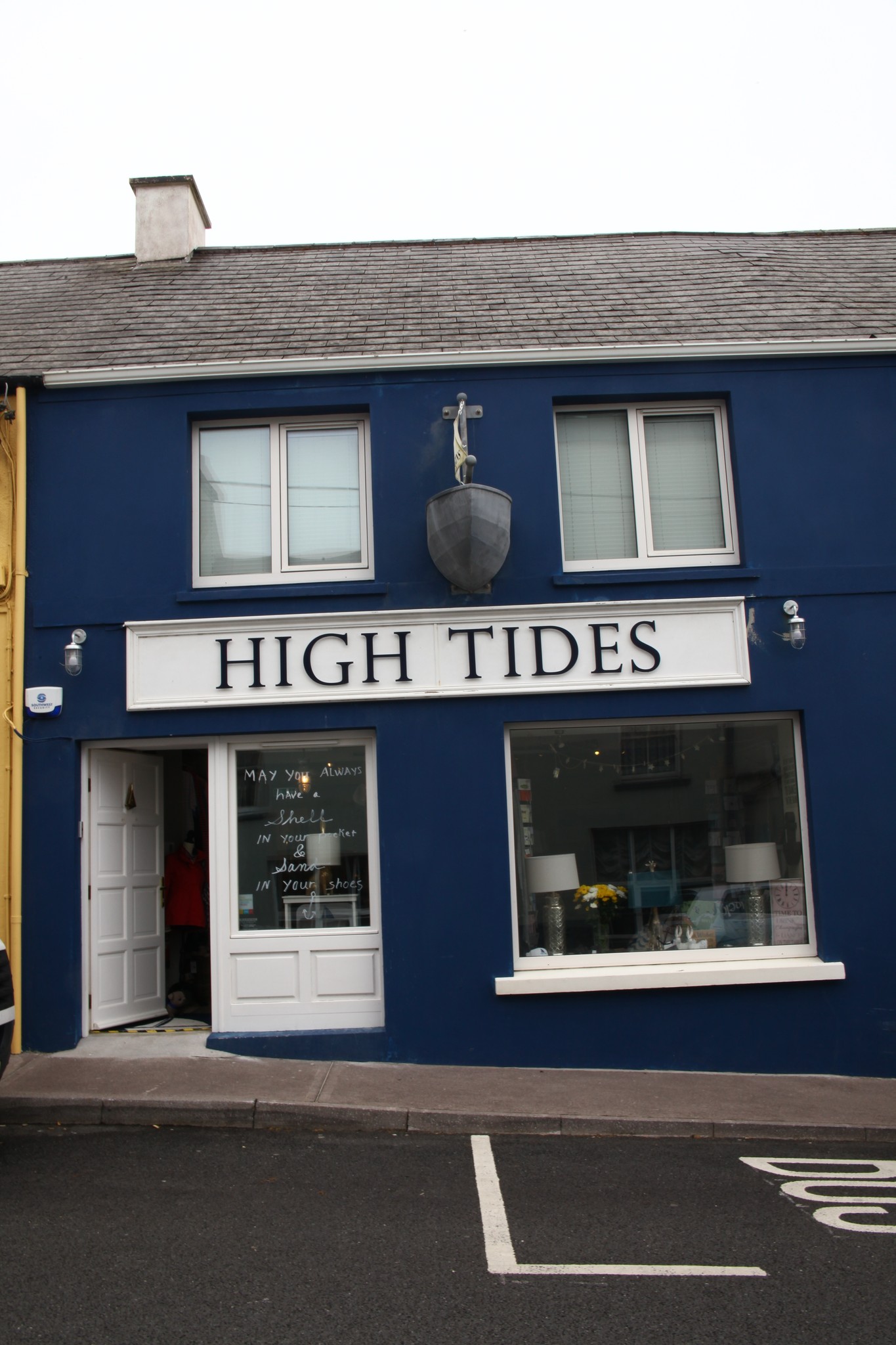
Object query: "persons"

[165,840,206,932]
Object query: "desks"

[281,893,359,931]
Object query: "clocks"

[767,877,807,945]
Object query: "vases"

[586,903,613,954]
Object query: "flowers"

[571,882,629,952]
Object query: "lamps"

[628,858,678,951]
[304,817,342,928]
[526,851,581,954]
[725,841,782,947]
[297,767,312,794]
[782,599,807,650]
[64,628,86,676]
